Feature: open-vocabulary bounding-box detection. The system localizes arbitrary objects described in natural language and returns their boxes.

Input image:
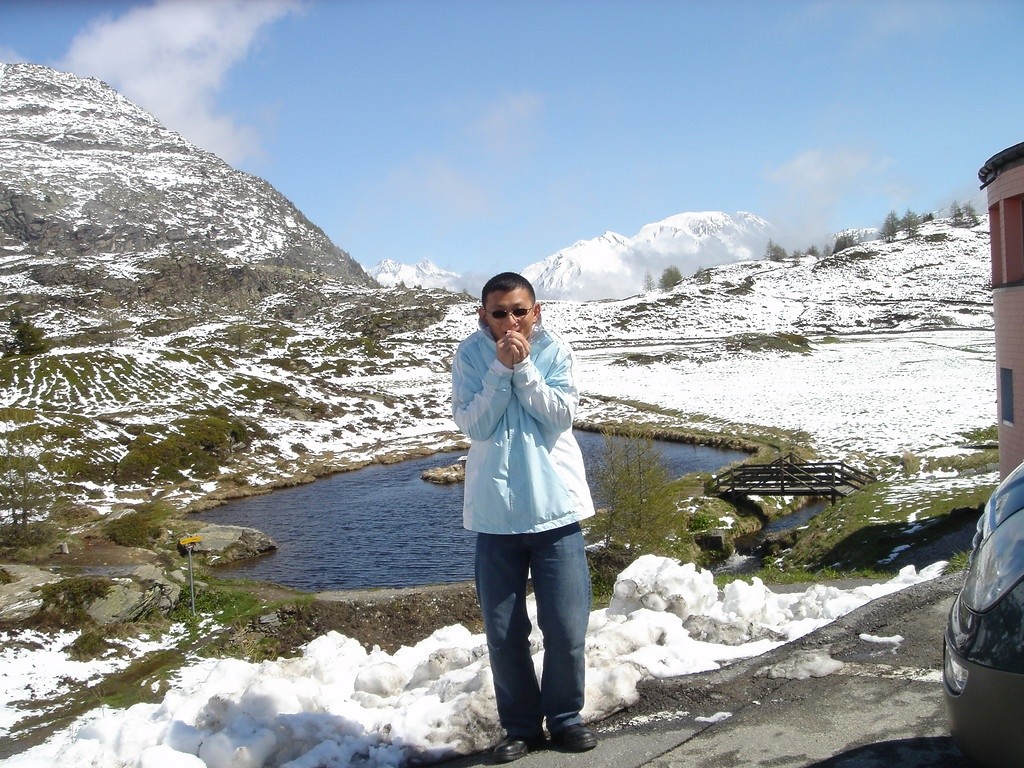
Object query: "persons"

[453,271,598,762]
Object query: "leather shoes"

[550,722,598,750]
[494,727,546,761]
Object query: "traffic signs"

[180,534,205,545]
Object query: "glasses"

[485,305,534,319]
